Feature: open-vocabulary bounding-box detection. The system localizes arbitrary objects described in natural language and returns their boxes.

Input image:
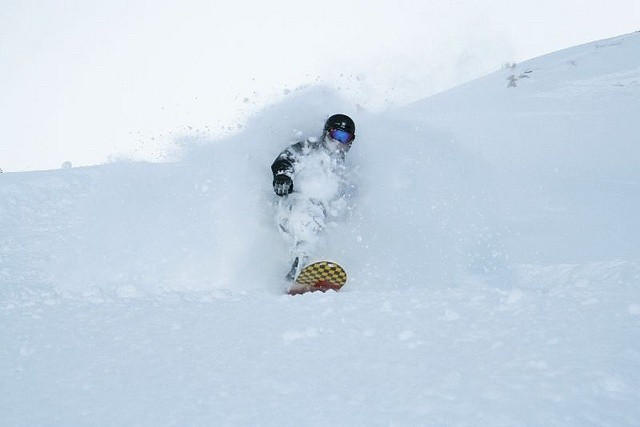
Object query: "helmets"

[326,114,355,135]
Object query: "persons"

[271,114,356,282]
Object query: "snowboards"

[288,260,347,296]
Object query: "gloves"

[272,173,292,196]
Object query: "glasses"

[326,129,354,144]
